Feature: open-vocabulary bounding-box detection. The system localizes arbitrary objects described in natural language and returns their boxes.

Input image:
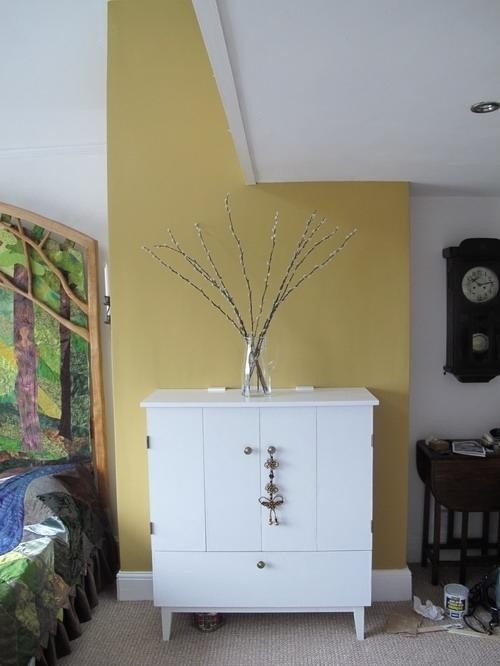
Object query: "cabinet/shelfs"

[138,387,384,643]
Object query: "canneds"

[443,583,469,620]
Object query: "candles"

[104,263,109,297]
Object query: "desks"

[416,438,499,587]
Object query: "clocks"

[442,238,500,384]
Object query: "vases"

[239,333,273,397]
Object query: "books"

[452,440,486,457]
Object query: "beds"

[1,454,108,666]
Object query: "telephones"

[481,427,500,454]
